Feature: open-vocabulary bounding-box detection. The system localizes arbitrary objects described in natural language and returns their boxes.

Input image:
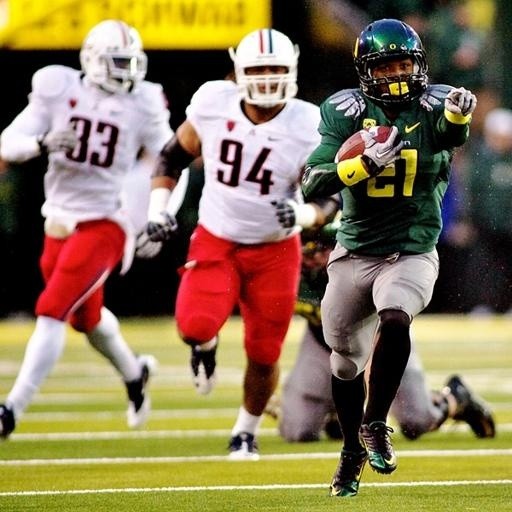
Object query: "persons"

[145,29,344,460]
[3,1,511,322]
[275,195,498,445]
[302,15,476,497]
[1,20,191,448]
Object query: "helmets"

[81,20,147,94]
[234,29,297,108]
[353,20,429,103]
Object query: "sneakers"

[357,421,400,473]
[191,336,220,393]
[230,430,258,461]
[330,446,366,497]
[443,377,494,437]
[0,399,17,441]
[124,355,156,424]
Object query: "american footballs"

[334,126,400,165]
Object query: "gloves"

[361,126,405,173]
[271,199,316,230]
[136,226,163,257]
[446,86,478,118]
[146,188,179,241]
